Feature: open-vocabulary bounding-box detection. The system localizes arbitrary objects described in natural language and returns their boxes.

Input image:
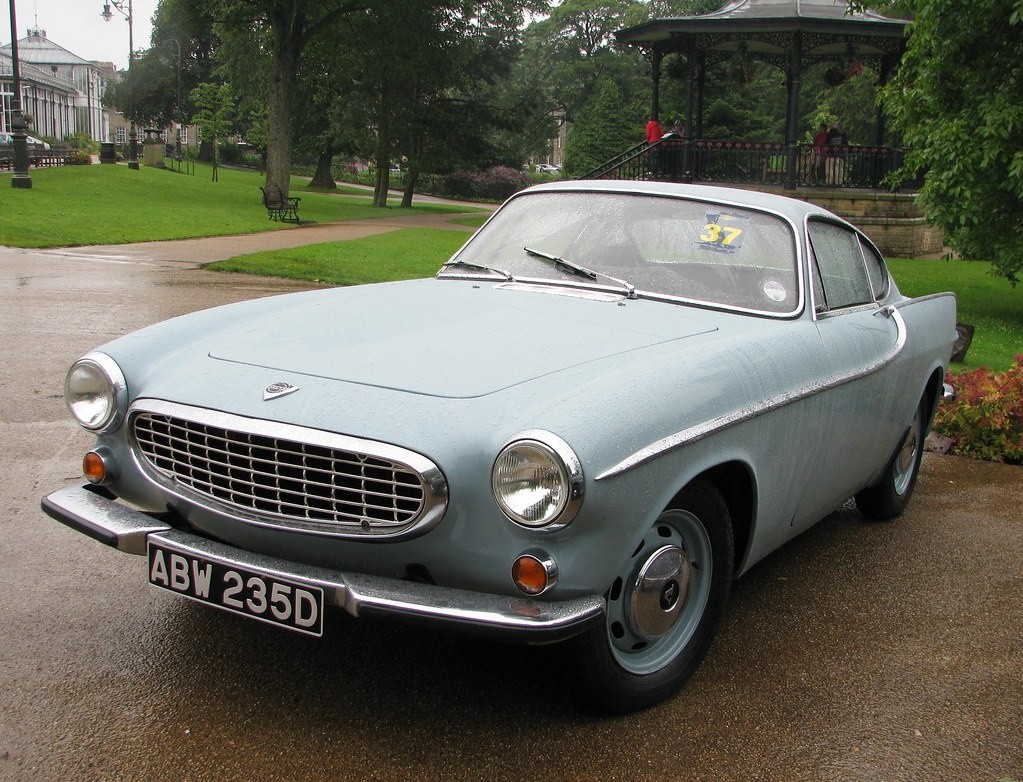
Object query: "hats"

[674,120,681,125]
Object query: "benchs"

[260,184,300,221]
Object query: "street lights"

[99,0,139,170]
[161,39,182,163]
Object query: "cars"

[0,132,51,164]
[634,169,694,181]
[42,182,959,710]
[345,163,369,175]
[522,163,562,176]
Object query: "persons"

[809,122,848,185]
[645,115,665,146]
